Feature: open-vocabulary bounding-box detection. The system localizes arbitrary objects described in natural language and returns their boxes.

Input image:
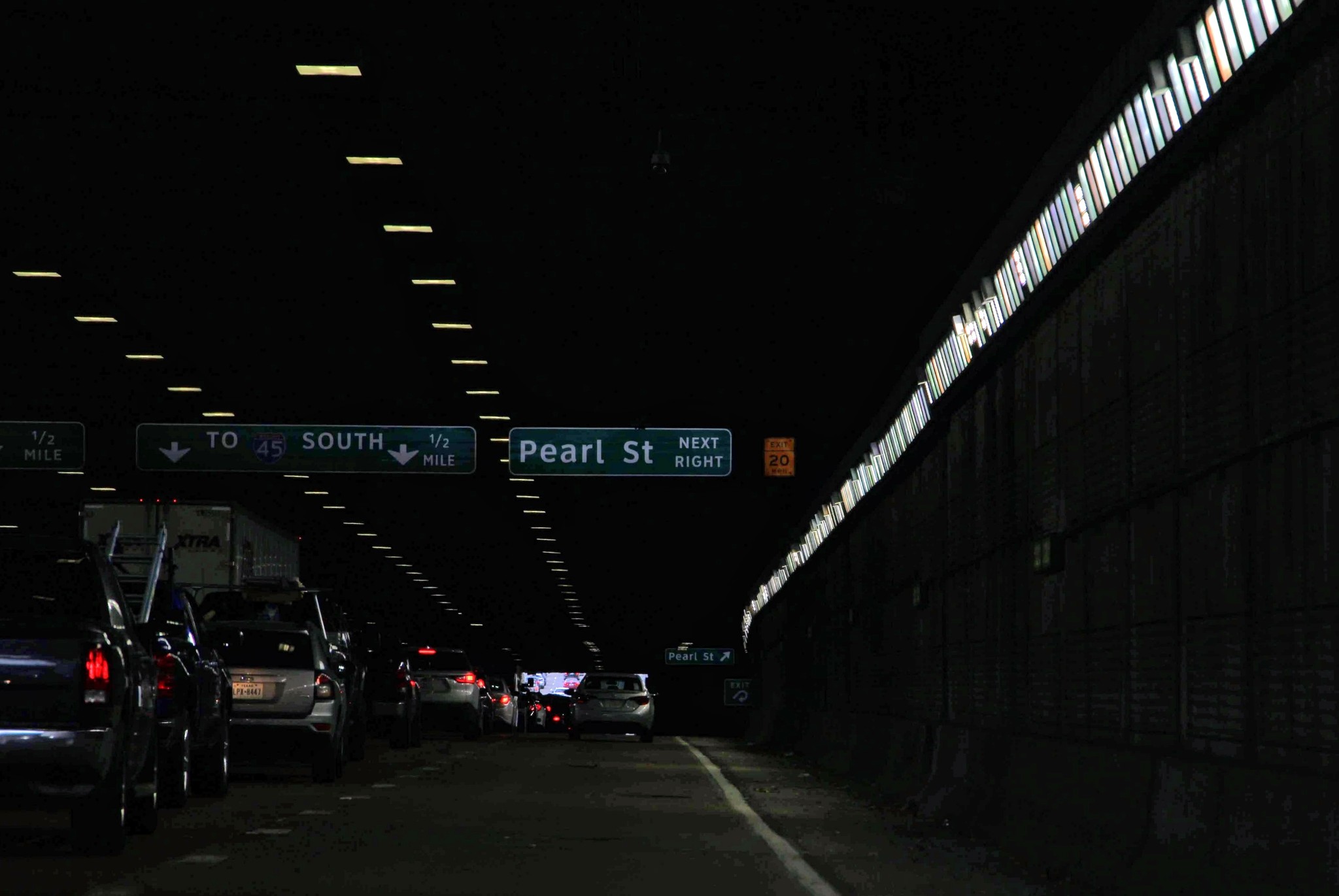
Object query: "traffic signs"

[665,648,735,666]
[135,423,477,473]
[509,427,731,476]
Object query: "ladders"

[105,520,169,623]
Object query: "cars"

[367,649,421,747]
[118,578,233,810]
[205,619,348,781]
[410,647,572,740]
[196,587,371,759]
[565,674,660,744]
[1,534,172,855]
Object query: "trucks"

[75,497,307,632]
